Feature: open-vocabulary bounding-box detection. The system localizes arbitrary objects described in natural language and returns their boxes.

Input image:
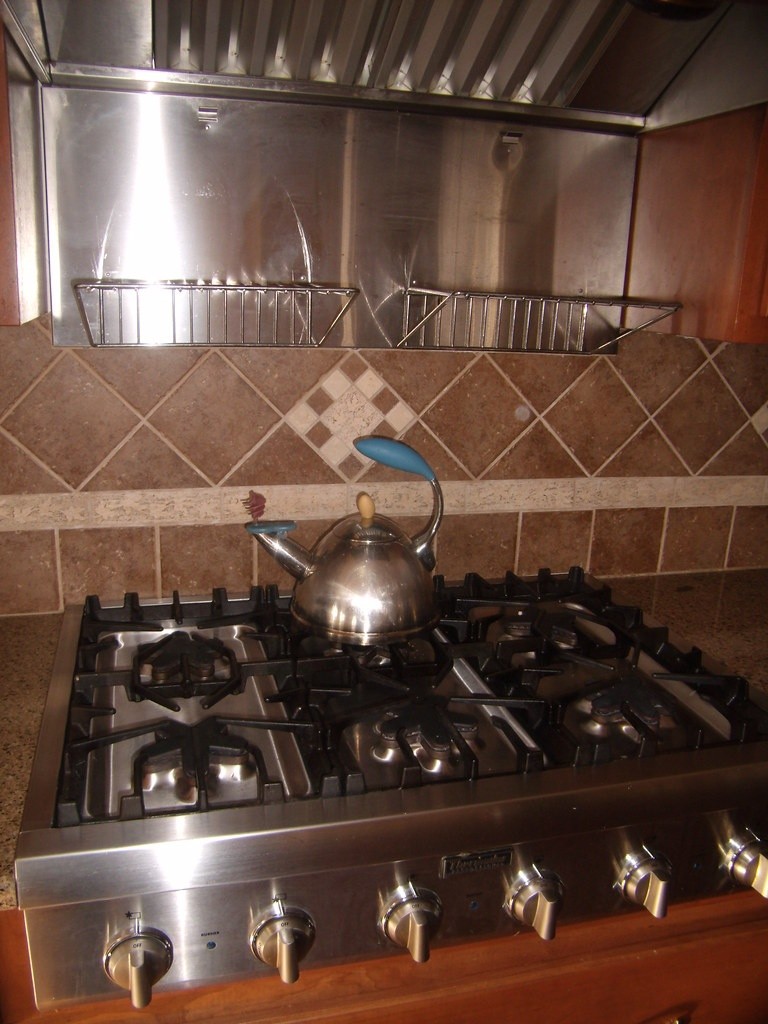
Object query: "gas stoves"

[13,562,767,1009]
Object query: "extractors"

[0,0,768,358]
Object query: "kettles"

[245,434,445,644]
